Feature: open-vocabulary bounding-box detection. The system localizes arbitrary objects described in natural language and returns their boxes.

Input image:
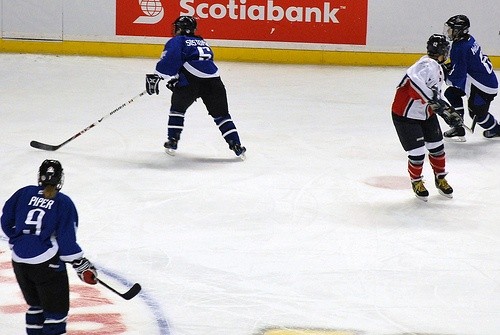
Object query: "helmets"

[171,16,197,35]
[443,15,470,40]
[427,34,449,61]
[38,159,63,191]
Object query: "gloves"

[73,257,97,285]
[145,74,163,95]
[442,106,464,126]
[165,78,179,91]
[428,99,446,114]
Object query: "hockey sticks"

[444,109,477,133]
[30,88,147,151]
[97,277,143,300]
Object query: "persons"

[0,159,98,335]
[391,14,500,203]
[146,15,246,160]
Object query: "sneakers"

[411,176,429,201]
[444,126,466,142]
[164,140,177,155]
[232,144,247,160]
[483,123,500,139]
[435,171,453,198]
